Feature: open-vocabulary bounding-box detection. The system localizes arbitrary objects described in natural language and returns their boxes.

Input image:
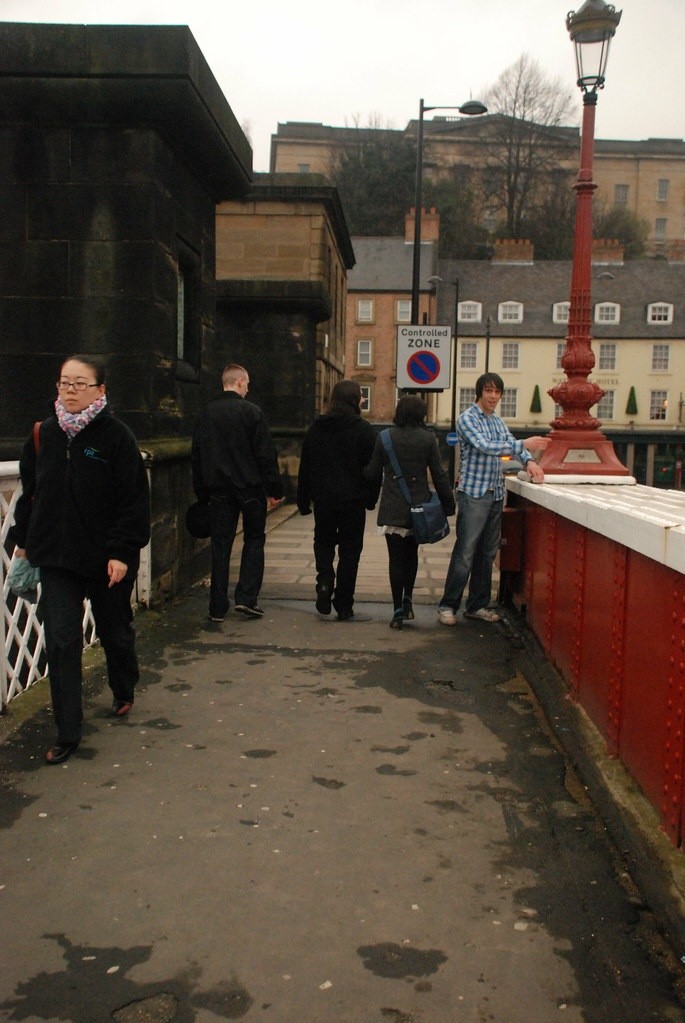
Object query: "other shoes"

[45,735,82,764]
[207,608,224,622]
[111,672,139,716]
[234,595,264,617]
[463,607,500,621]
[439,606,456,625]
[338,607,354,619]
[316,585,332,615]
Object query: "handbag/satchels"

[410,494,450,544]
[23,422,42,560]
[186,495,213,539]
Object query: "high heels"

[390,611,404,631]
[403,597,414,619]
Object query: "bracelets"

[526,458,535,466]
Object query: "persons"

[436,373,551,624]
[297,381,382,619]
[191,365,286,622]
[365,396,456,628]
[16,351,150,764]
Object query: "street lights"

[409,96,491,325]
[516,0,638,488]
[426,275,460,489]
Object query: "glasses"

[56,381,102,392]
